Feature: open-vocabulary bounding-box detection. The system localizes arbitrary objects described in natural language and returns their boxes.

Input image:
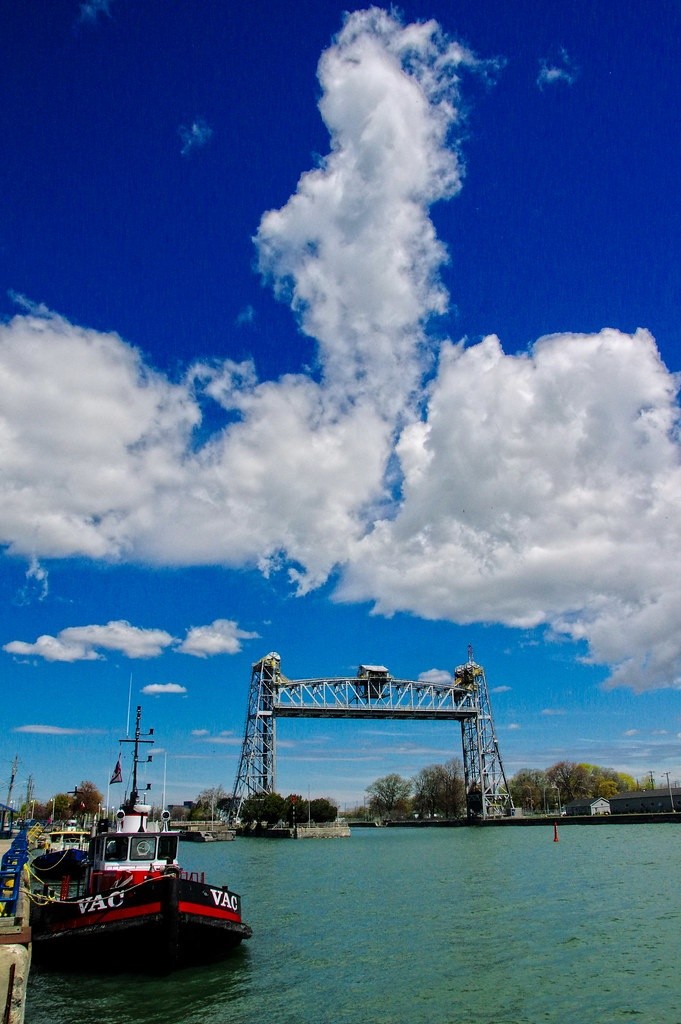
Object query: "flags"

[109,753,122,784]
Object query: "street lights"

[102,808,106,819]
[98,802,101,821]
[50,798,55,821]
[9,800,14,809]
[112,806,115,822]
[143,793,147,805]
[664,771,675,812]
[512,785,563,817]
[30,799,35,819]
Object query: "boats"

[30,707,252,978]
[29,820,91,879]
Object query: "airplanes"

[66,785,84,796]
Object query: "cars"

[2,817,80,831]
